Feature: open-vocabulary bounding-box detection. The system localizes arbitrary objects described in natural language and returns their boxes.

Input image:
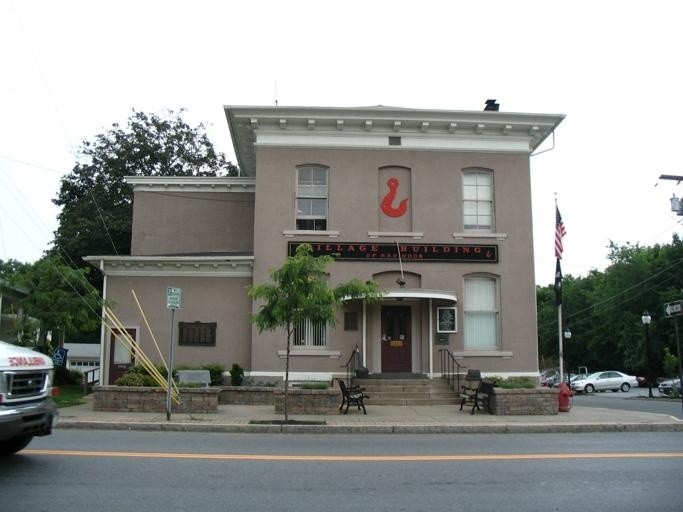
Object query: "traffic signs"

[663,300,681,319]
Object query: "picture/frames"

[435,305,458,334]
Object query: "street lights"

[639,309,654,398]
[562,328,572,388]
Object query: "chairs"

[457,377,498,414]
[335,378,369,415]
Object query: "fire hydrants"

[557,383,572,414]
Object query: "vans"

[0,339,58,459]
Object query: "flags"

[554,208,568,259]
[553,258,564,307]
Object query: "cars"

[658,375,681,398]
[540,368,646,393]
